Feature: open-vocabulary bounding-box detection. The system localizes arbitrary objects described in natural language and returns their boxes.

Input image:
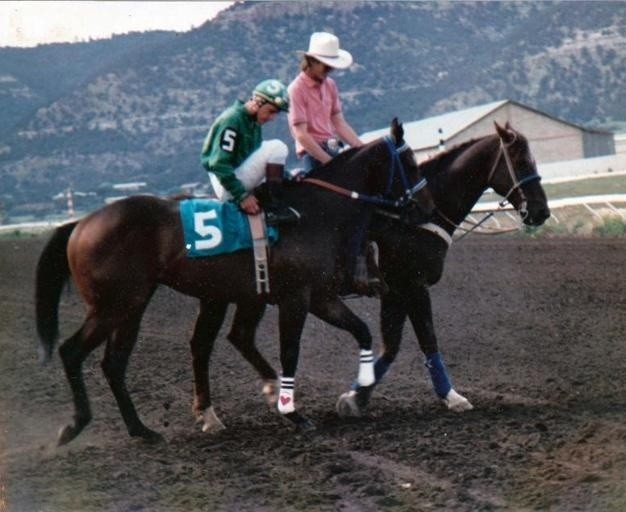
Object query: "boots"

[264,165,302,225]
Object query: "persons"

[285,31,366,174]
[200,78,311,227]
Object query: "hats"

[293,33,353,70]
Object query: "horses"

[33,115,439,448]
[188,118,551,435]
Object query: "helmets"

[252,79,290,111]
